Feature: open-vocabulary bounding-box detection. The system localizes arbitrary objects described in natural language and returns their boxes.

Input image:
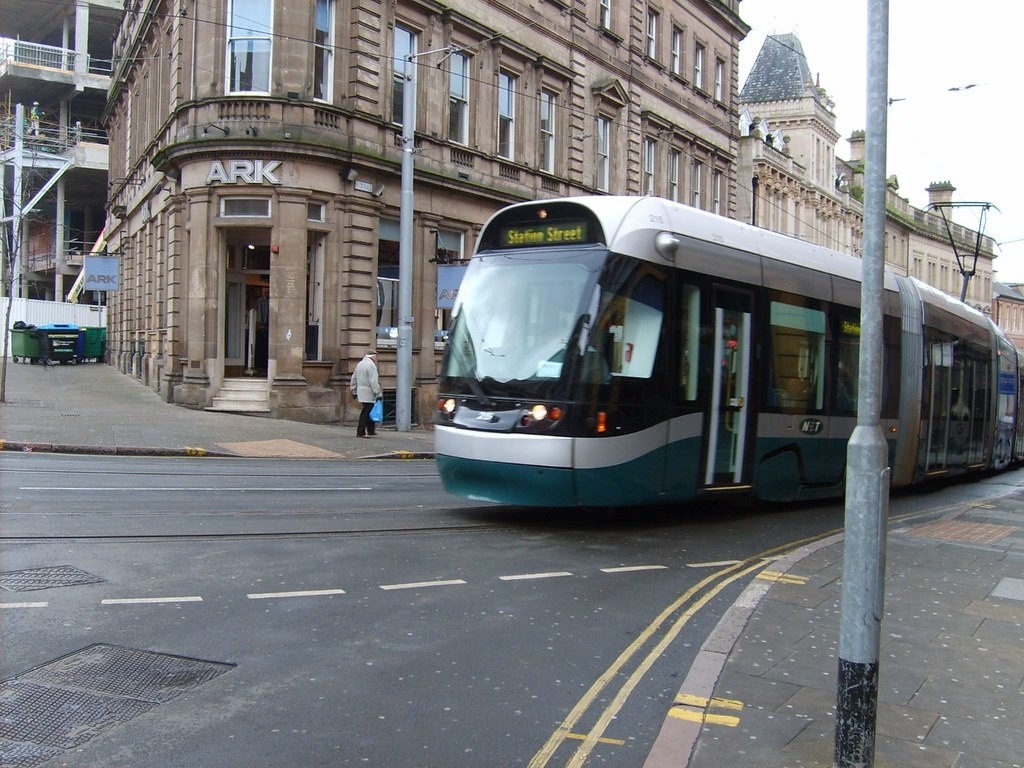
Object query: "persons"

[26,102,45,136]
[349,349,383,438]
[567,344,610,402]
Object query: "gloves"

[376,396,383,402]
[352,395,357,400]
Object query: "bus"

[434,196,1024,508]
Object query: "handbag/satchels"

[369,400,384,422]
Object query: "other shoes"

[367,431,378,435]
[357,434,371,439]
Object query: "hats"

[367,349,379,355]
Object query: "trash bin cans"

[37,324,80,366]
[9,329,40,364]
[80,325,106,364]
